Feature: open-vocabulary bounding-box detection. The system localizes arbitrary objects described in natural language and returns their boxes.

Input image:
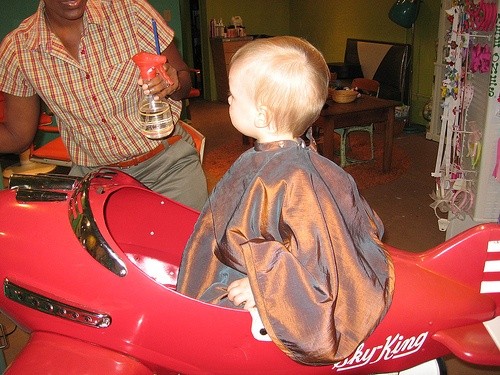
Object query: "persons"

[0,0,208,212]
[176,36,397,365]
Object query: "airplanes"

[0,160,500,375]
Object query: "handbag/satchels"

[388,0,419,28]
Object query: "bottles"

[224,26,227,38]
[237,23,246,37]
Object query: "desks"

[311,90,402,172]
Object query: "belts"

[109,135,182,169]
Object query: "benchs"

[327,38,412,103]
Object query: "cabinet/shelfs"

[210,36,253,100]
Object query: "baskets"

[327,86,356,104]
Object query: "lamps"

[388,0,424,134]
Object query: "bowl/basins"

[333,90,359,103]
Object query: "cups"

[227,28,237,37]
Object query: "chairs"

[315,78,380,167]
